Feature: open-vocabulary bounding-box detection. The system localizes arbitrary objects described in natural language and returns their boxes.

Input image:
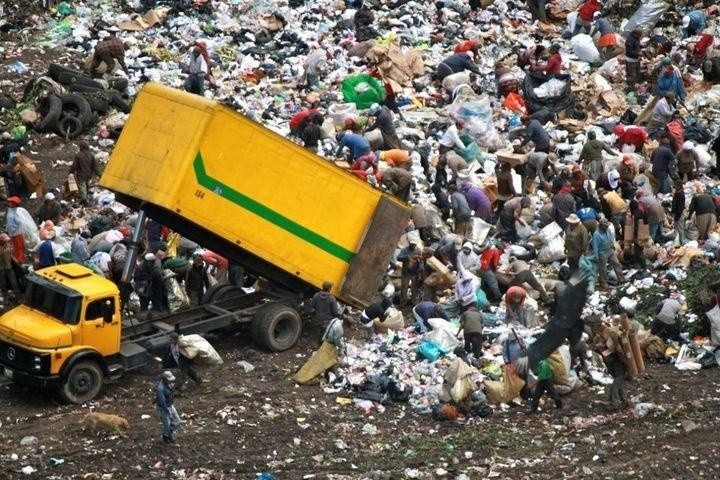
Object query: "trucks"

[2,81,414,405]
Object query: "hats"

[409,234,420,244]
[145,252,156,260]
[547,131,694,226]
[368,103,383,115]
[162,371,176,382]
[7,196,21,204]
[322,281,334,290]
[0,233,10,241]
[45,193,55,200]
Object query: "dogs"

[77,412,130,439]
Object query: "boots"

[162,433,174,444]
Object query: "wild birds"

[513,265,593,379]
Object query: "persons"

[90,36,132,75]
[303,40,329,86]
[153,371,181,444]
[158,333,202,384]
[290,108,324,152]
[181,41,209,96]
[0,140,229,312]
[335,0,720,415]
[311,281,337,344]
